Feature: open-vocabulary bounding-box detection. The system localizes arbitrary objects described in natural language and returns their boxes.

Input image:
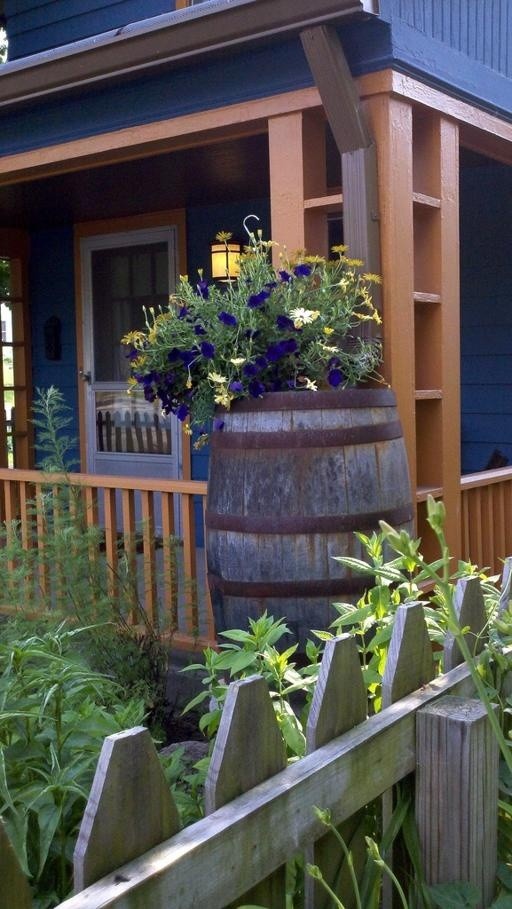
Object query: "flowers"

[128,223,387,448]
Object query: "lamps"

[208,237,240,286]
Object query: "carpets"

[94,531,168,558]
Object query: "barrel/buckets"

[205,388,417,707]
